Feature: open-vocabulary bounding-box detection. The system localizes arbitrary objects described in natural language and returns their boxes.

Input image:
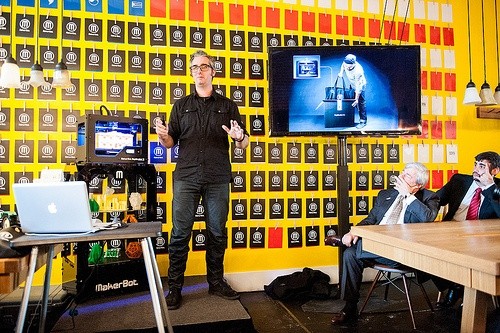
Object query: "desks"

[8,221,173,333]
[350,218,500,333]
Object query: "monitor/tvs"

[267,43,421,135]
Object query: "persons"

[154,50,250,309]
[338,55,367,129]
[435,152,500,311]
[325,162,440,326]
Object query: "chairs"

[359,262,435,330]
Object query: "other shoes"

[356,122,365,129]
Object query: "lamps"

[475,0,497,106]
[463,0,482,105]
[0,0,23,88]
[493,0,500,105]
[28,0,47,87]
[52,0,72,89]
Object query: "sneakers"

[165,287,182,309]
[208,279,239,299]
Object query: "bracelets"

[237,134,246,142]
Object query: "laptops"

[12,180,118,232]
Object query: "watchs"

[404,193,412,198]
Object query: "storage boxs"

[0,284,72,333]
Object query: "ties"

[386,195,404,225]
[466,188,481,221]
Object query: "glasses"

[188,64,212,72]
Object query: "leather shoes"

[330,311,358,326]
[444,287,463,307]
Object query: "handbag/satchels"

[325,77,355,100]
[264,269,330,306]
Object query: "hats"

[344,54,356,65]
